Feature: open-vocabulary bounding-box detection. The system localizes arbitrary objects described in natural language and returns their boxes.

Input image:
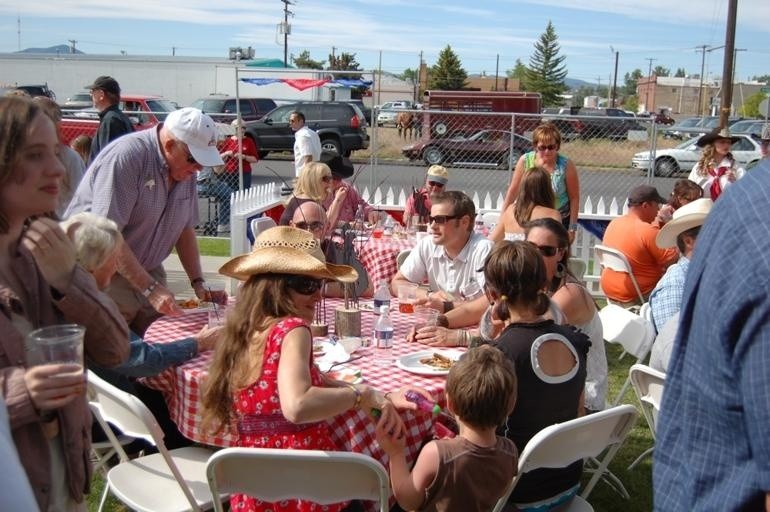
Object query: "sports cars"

[402,128,536,170]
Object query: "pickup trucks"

[549,108,648,143]
[60,95,234,167]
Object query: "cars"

[663,117,703,141]
[630,132,764,178]
[683,117,742,142]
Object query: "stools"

[195,194,220,237]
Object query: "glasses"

[180,147,197,166]
[236,126,247,130]
[320,175,333,184]
[293,220,328,231]
[428,182,444,187]
[288,119,299,123]
[533,142,560,151]
[286,276,324,295]
[527,240,566,256]
[427,215,465,226]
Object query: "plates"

[353,237,369,242]
[336,228,366,235]
[350,221,374,230]
[313,340,335,355]
[175,300,219,313]
[335,370,364,384]
[357,302,394,312]
[396,352,465,375]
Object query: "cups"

[372,226,384,238]
[398,227,408,240]
[28,323,85,379]
[208,307,224,327]
[398,283,418,312]
[416,231,427,244]
[415,309,439,341]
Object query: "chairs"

[494,404,638,511]
[594,244,647,362]
[206,446,392,512]
[630,364,666,472]
[615,303,657,403]
[85,369,230,511]
[251,216,276,240]
[568,258,587,282]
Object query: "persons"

[193,167,235,233]
[688,128,748,203]
[390,191,495,315]
[58,212,226,458]
[652,179,705,233]
[599,186,680,310]
[403,165,449,231]
[276,162,347,236]
[83,76,136,170]
[290,111,321,179]
[408,218,607,415]
[482,243,592,512]
[648,198,715,333]
[288,200,375,298]
[199,227,435,512]
[499,122,580,246]
[214,118,259,191]
[316,149,384,226]
[62,107,224,339]
[488,166,562,241]
[374,344,518,512]
[1,94,131,512]
[32,95,86,222]
[68,132,92,168]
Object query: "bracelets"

[455,329,471,348]
[190,277,205,289]
[346,383,363,414]
[141,280,160,298]
[568,223,578,233]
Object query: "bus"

[421,89,543,139]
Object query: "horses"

[395,104,418,140]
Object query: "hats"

[314,148,356,180]
[82,75,122,98]
[164,106,226,168]
[427,164,449,185]
[695,125,745,149]
[656,198,716,248]
[218,223,361,283]
[752,122,770,143]
[626,182,671,209]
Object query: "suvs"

[244,100,371,161]
[650,113,675,126]
[185,96,278,126]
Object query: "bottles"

[373,279,391,315]
[383,214,393,235]
[355,204,364,222]
[373,305,394,354]
